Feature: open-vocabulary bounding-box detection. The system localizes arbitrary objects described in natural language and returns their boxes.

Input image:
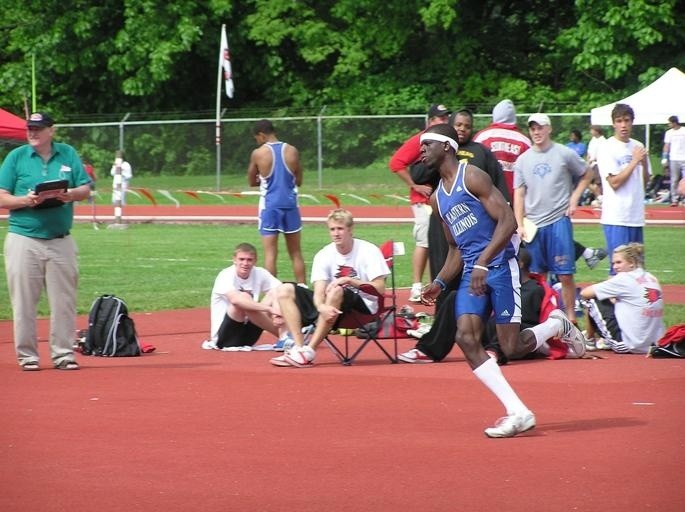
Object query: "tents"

[591,68,685,154]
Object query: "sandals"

[22,359,80,370]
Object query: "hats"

[26,112,54,128]
[527,113,551,127]
[429,104,452,118]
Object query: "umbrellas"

[0,109,27,140]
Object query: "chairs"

[314,240,398,366]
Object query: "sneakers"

[585,248,608,270]
[270,324,314,367]
[408,283,423,302]
[549,309,611,357]
[484,411,535,438]
[396,348,434,363]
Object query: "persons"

[246,120,304,287]
[419,124,588,437]
[0,112,93,371]
[269,207,393,366]
[392,99,607,365]
[597,103,652,279]
[588,126,606,164]
[81,158,99,205]
[207,242,296,350]
[579,243,664,351]
[645,115,685,206]
[109,148,132,205]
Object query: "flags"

[219,25,239,97]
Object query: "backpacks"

[81,295,140,357]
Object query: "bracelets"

[473,262,492,272]
[434,278,448,293]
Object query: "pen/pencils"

[33,198,38,204]
